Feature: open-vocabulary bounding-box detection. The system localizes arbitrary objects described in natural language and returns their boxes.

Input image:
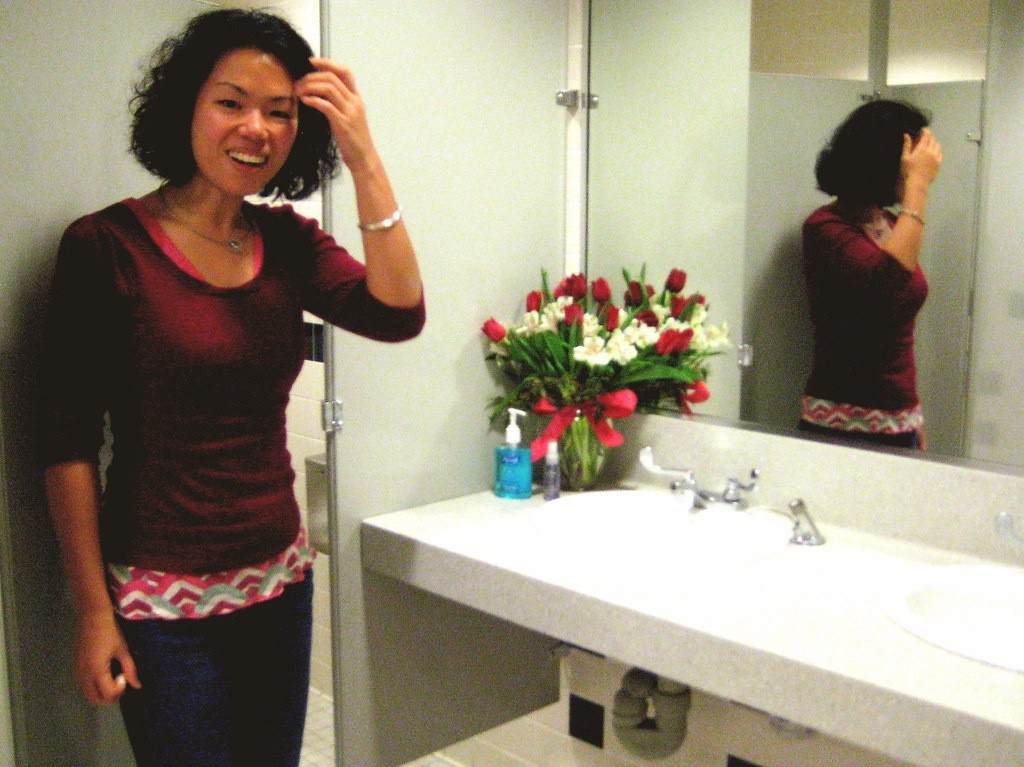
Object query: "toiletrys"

[489,406,534,500]
[542,436,561,499]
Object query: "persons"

[801,99,942,451]
[34,9,426,767]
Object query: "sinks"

[532,487,791,603]
[880,564,1023,672]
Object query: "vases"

[535,414,615,490]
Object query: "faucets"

[685,468,763,535]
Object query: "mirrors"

[578,0,1024,479]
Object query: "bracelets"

[358,205,401,232]
[898,209,925,225]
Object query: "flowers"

[480,265,707,460]
[621,262,733,416]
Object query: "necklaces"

[864,224,885,237]
[158,186,253,252]
